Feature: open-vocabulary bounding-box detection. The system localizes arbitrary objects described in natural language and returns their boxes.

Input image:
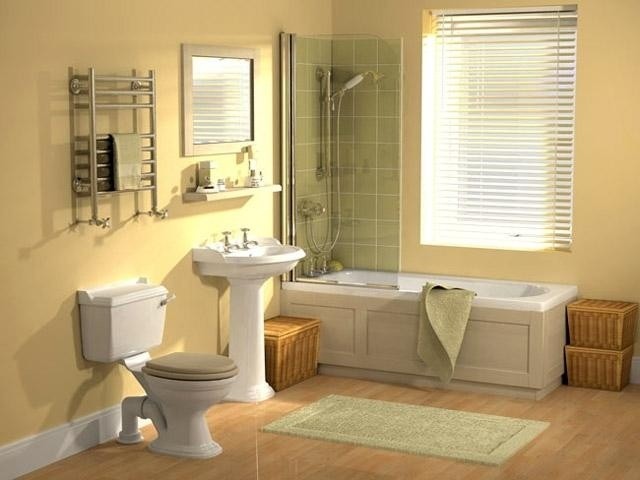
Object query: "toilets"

[77,280,238,459]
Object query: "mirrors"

[181,44,259,157]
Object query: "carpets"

[259,392,550,467]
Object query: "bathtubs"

[281,271,578,313]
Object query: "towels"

[95,133,141,194]
[417,282,477,389]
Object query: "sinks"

[192,237,306,277]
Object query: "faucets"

[222,232,242,251]
[241,228,258,248]
